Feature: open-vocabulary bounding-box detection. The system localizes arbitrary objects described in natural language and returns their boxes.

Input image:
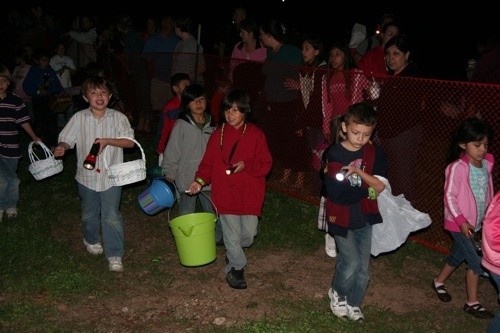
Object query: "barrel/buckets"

[136,177,177,216]
[167,189,218,267]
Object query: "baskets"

[27,141,63,181]
[103,137,147,186]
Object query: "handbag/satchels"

[370,190,433,257]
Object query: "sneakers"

[107,256,123,272]
[346,304,365,322]
[327,287,347,316]
[83,238,103,255]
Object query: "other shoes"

[432,280,452,301]
[463,302,494,318]
[227,267,248,288]
[0,208,18,220]
[325,234,336,258]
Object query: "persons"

[480,191,500,333]
[186,90,272,289]
[53,76,135,272]
[0,1,500,250]
[432,118,495,319]
[161,84,225,246]
[319,101,387,322]
